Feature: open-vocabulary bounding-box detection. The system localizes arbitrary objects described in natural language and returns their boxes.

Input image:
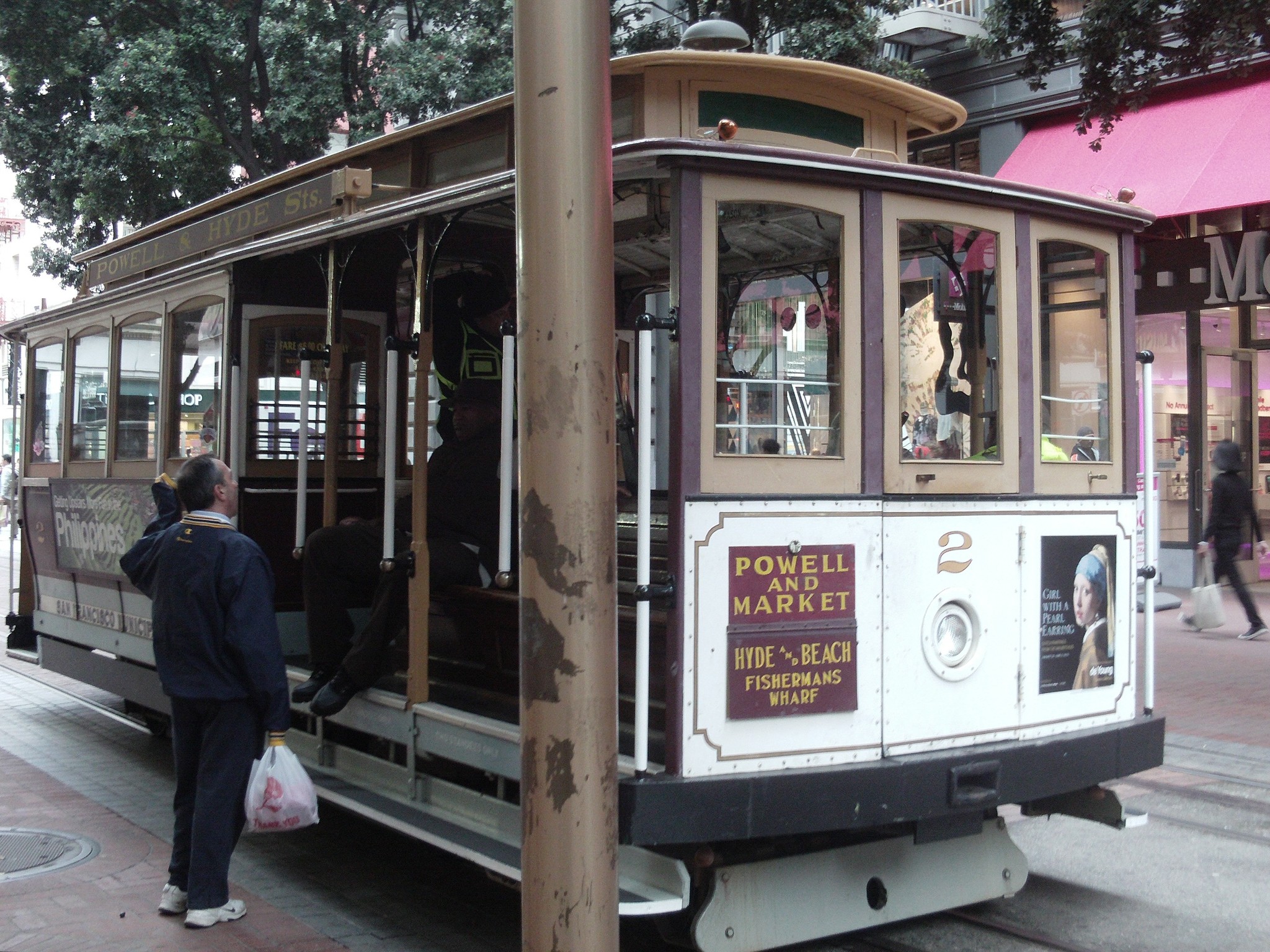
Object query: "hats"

[1077,427,1094,443]
[438,378,500,408]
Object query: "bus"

[0,23,1176,939]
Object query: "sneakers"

[1179,612,1201,632]
[156,883,188,914]
[309,672,357,716]
[1238,623,1269,639]
[290,670,336,703]
[184,899,248,928]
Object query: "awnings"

[914,65,1270,274]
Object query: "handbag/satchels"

[1191,553,1225,629]
[244,743,319,833]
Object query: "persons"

[958,400,1069,462]
[0,454,19,539]
[1070,427,1101,461]
[120,456,290,928]
[762,439,781,454]
[1072,544,1115,689]
[294,259,513,713]
[1175,439,1270,640]
